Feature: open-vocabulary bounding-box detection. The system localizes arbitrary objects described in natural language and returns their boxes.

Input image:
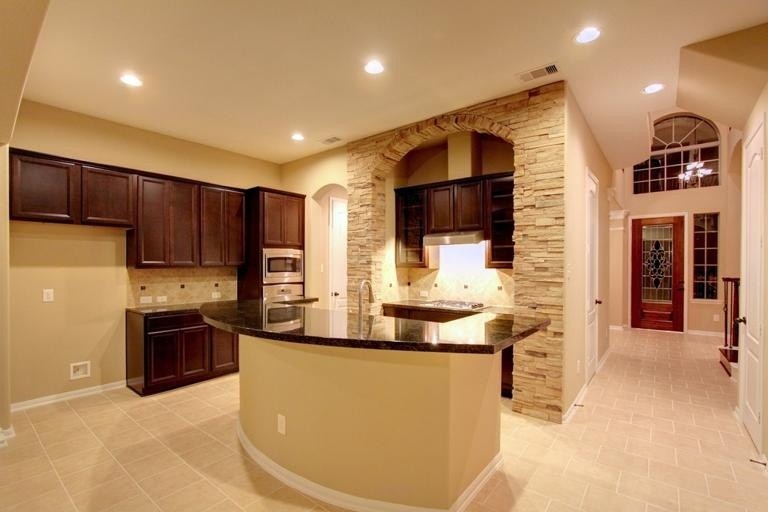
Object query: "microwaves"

[263,301,303,332]
[263,248,303,284]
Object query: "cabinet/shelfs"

[9,147,306,268]
[126,300,249,396]
[382,304,512,400]
[393,171,513,270]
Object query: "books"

[496,310,514,321]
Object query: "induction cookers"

[417,299,491,311]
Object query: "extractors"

[422,231,484,246]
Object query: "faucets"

[349,279,375,314]
[347,314,374,341]
